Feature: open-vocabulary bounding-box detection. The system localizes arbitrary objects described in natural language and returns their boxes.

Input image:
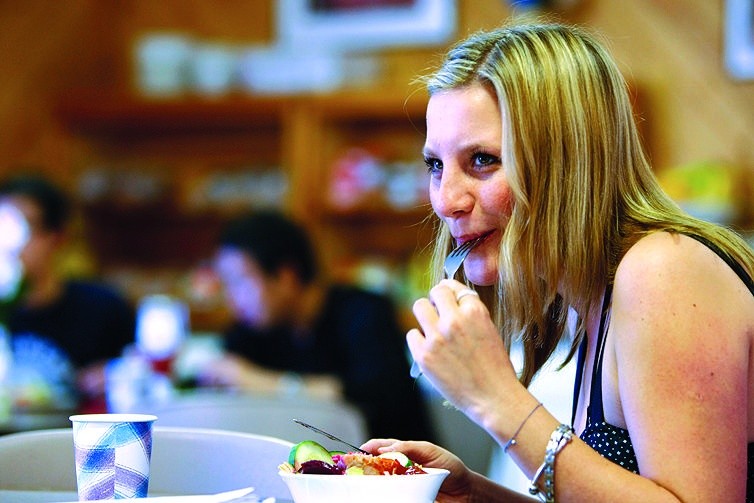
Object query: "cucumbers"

[288,441,413,470]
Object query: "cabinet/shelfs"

[51,78,447,338]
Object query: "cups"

[70,413,158,503]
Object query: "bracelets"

[528,428,571,494]
[538,424,574,501]
[504,404,543,452]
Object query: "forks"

[409,230,496,378]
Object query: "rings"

[456,290,479,302]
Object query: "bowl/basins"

[280,467,451,503]
[0,427,300,502]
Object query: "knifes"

[292,419,372,456]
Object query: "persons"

[0,1,753,502]
